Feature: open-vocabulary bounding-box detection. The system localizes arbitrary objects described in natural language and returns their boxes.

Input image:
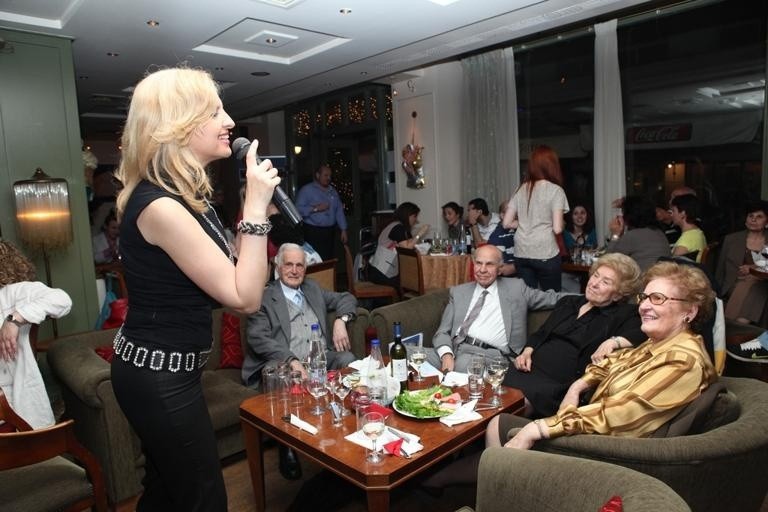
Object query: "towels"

[410,361,468,386]
[345,424,423,455]
[439,399,483,427]
[349,355,380,371]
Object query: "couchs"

[369,290,552,355]
[41,306,367,512]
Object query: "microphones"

[233,137,303,227]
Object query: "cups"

[261,362,305,407]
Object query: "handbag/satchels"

[95,270,128,331]
[354,242,376,282]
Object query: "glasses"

[637,292,689,305]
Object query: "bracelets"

[236,217,274,236]
[312,205,318,213]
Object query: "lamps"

[13,169,74,349]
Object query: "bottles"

[458,217,471,254]
[369,322,409,398]
[306,326,329,368]
[572,245,599,262]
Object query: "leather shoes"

[279,447,301,479]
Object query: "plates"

[391,387,459,421]
[342,370,367,390]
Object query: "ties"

[453,291,488,356]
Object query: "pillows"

[95,346,114,363]
[218,312,242,369]
[598,495,622,512]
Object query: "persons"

[296,165,348,292]
[0,280,73,429]
[109,59,282,512]
[405,145,768,451]
[366,201,432,287]
[238,185,308,246]
[239,242,359,482]
[212,184,231,227]
[93,213,122,275]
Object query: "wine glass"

[422,232,468,255]
[306,368,353,428]
[466,351,509,406]
[350,384,386,465]
[410,344,427,382]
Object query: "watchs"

[6,312,22,327]
[336,315,348,323]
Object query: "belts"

[462,336,495,349]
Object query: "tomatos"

[448,399,455,404]
[435,393,442,398]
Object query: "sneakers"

[727,340,768,363]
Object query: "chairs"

[0,237,37,358]
[305,258,336,293]
[0,388,109,512]
[658,255,761,369]
[508,377,768,512]
[344,246,397,303]
[455,446,692,512]
[396,247,423,301]
[701,241,722,282]
[105,273,126,300]
